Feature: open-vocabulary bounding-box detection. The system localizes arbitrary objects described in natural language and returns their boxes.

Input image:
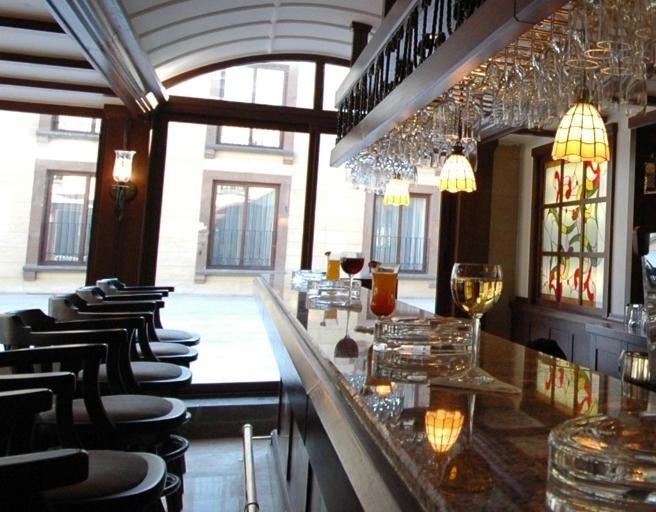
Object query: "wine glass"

[335,310,359,358]
[339,251,365,308]
[449,263,503,383]
[344,1,656,195]
[441,391,494,495]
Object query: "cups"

[327,252,341,282]
[370,264,401,319]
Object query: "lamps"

[550,68,612,164]
[384,171,411,207]
[111,150,136,219]
[437,112,478,194]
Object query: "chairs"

[0,273,199,511]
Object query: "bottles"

[629,304,640,327]
